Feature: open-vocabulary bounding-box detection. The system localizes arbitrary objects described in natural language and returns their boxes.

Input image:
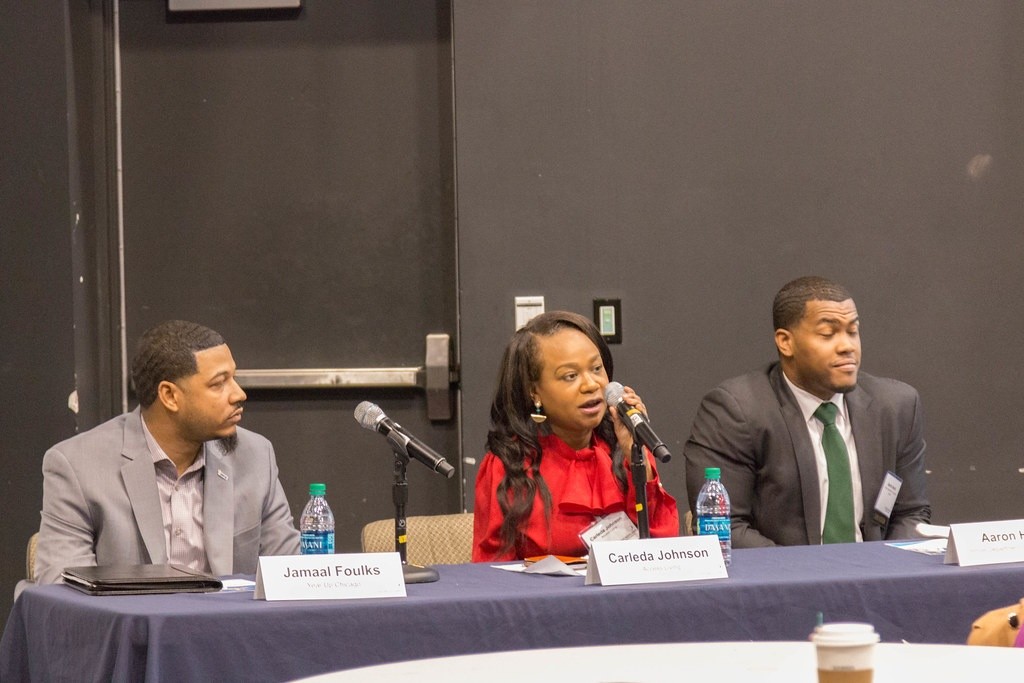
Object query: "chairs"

[360,514,474,566]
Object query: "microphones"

[353,401,456,480]
[604,381,671,464]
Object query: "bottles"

[696,468,732,567]
[299,483,335,555]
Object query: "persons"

[470,309,681,564]
[30,318,302,584]
[682,274,933,549]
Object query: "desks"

[0,540,1024,682]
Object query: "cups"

[810,622,881,683]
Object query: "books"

[61,563,223,596]
